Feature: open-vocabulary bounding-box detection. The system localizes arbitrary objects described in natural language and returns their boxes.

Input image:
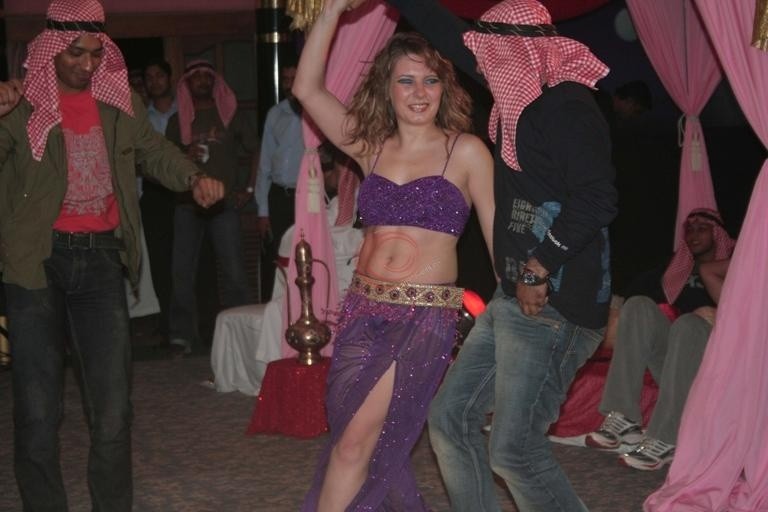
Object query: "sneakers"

[162,341,195,360]
[584,410,645,450]
[618,440,678,472]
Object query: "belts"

[54,232,124,250]
[272,183,296,198]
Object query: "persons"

[0,0,231,510]
[1,55,364,368]
[422,0,627,510]
[287,1,504,510]
[581,206,733,472]
[594,80,653,130]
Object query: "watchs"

[517,268,546,287]
